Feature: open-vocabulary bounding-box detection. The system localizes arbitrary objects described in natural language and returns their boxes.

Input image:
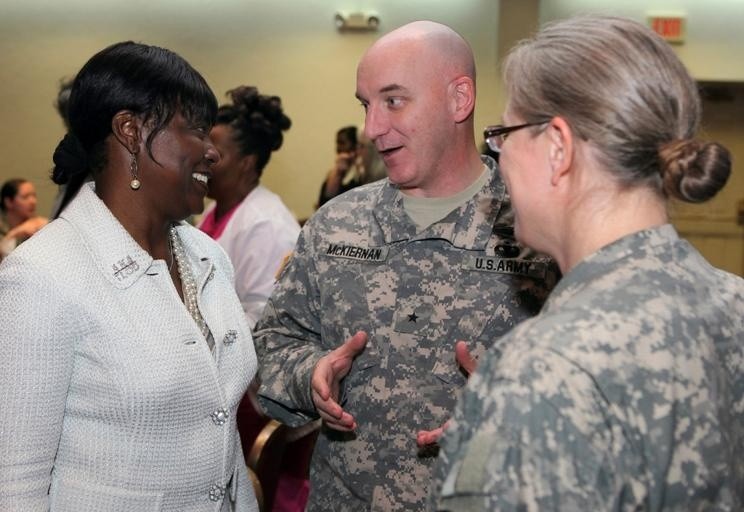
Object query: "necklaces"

[165,226,208,338]
[168,239,174,271]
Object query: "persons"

[319,127,367,207]
[324,130,388,200]
[427,15,744,512]
[252,18,562,512]
[195,86,312,512]
[0,179,50,264]
[1,41,260,512]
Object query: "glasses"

[482,121,542,153]
